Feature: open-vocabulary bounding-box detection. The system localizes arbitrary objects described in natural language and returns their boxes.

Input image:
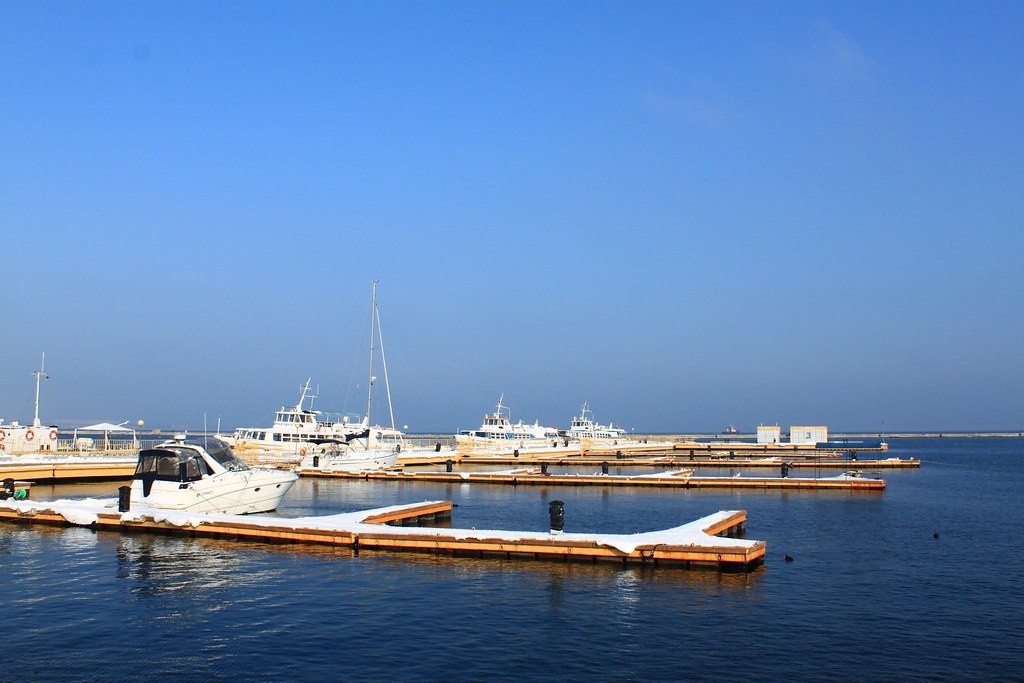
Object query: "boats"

[455,394,582,449]
[722,424,740,434]
[569,401,625,439]
[127,413,299,513]
[301,439,402,470]
[214,417,236,448]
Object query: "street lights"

[404,425,408,447]
[138,420,144,448]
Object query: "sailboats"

[214,280,405,453]
